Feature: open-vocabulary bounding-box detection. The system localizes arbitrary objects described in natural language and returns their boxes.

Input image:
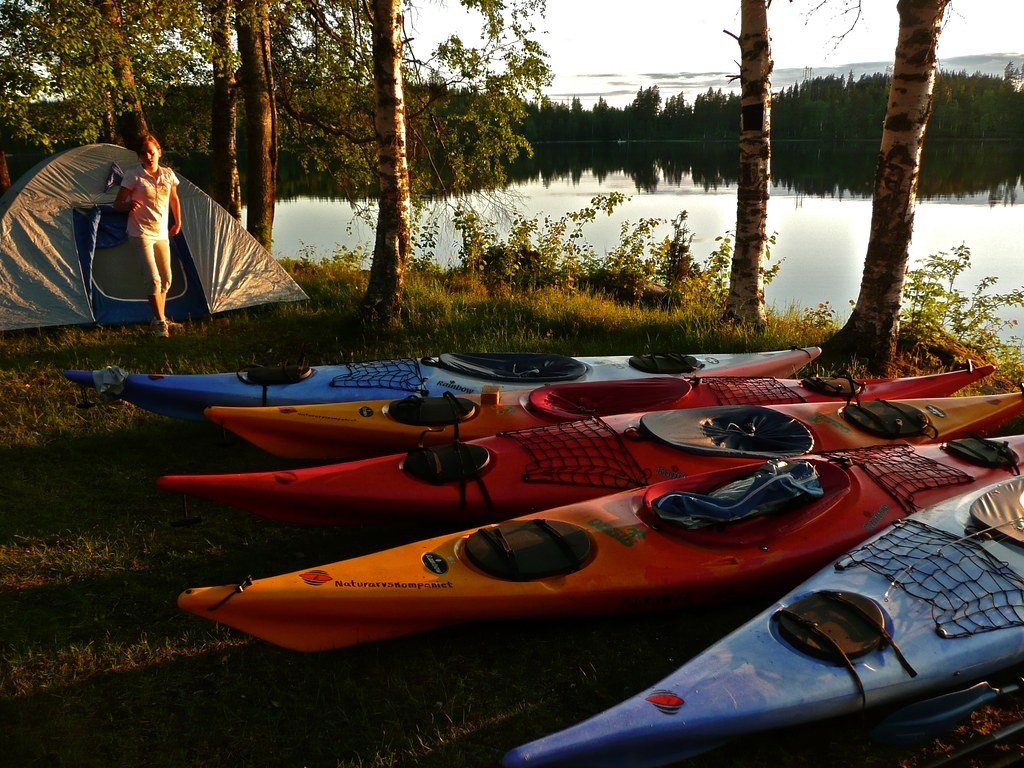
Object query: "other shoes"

[156,321,169,338]
[166,321,183,329]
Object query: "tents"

[0,142,311,332]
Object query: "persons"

[113,128,183,338]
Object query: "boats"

[61,348,1024,768]
